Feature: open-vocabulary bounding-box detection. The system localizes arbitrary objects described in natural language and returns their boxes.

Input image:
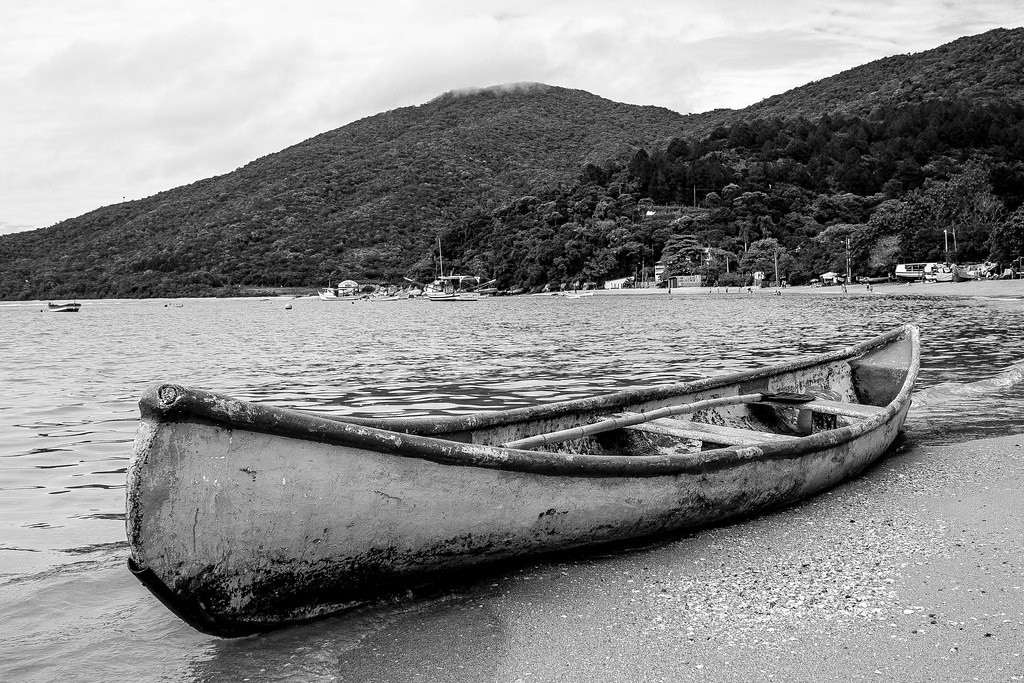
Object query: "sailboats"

[424,237,481,301]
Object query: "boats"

[318,278,369,300]
[124,323,919,638]
[47,301,81,313]
